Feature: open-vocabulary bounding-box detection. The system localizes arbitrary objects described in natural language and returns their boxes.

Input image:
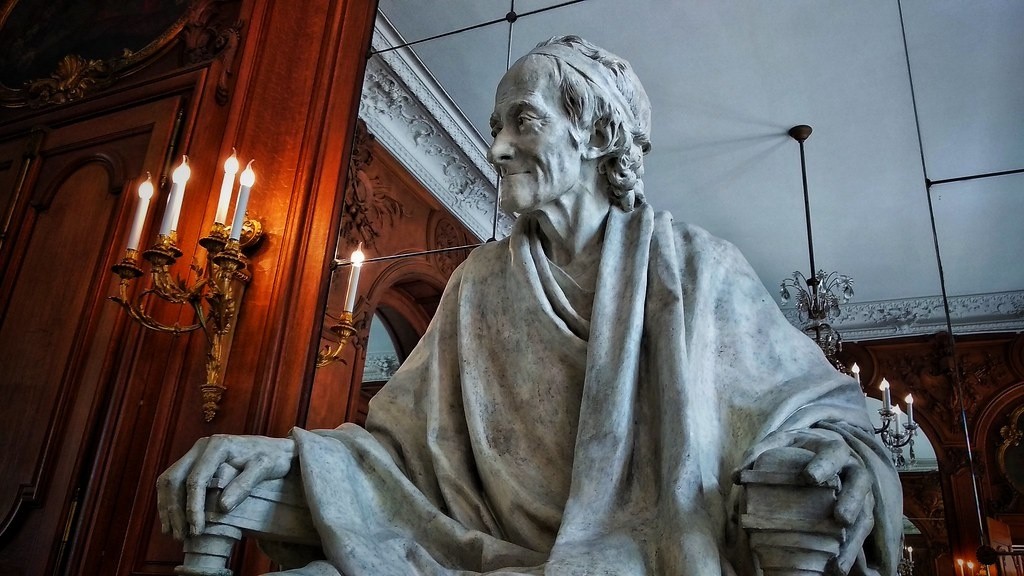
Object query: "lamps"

[314,243,365,367]
[107,147,266,423]
[779,125,920,470]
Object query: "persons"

[154,33,905,576]
